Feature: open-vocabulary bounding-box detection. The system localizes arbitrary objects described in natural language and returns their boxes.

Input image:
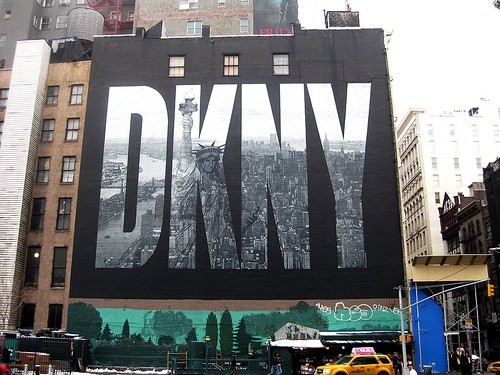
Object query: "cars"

[313,346,395,375]
[487,361,500,374]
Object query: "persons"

[448,351,473,375]
[176,114,230,270]
[266,353,280,375]
[261,347,268,359]
[0,334,11,366]
[407,363,418,375]
[338,354,344,364]
[248,350,259,359]
[392,352,402,375]
